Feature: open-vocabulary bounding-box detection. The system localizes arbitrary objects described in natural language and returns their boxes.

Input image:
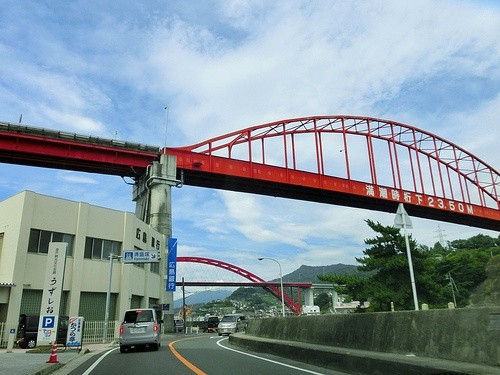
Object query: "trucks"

[185,316,222,330]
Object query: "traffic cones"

[45,341,61,364]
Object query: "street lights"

[257,257,285,317]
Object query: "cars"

[217,314,249,336]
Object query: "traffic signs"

[122,250,159,264]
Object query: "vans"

[119,309,164,353]
[14,313,72,349]
